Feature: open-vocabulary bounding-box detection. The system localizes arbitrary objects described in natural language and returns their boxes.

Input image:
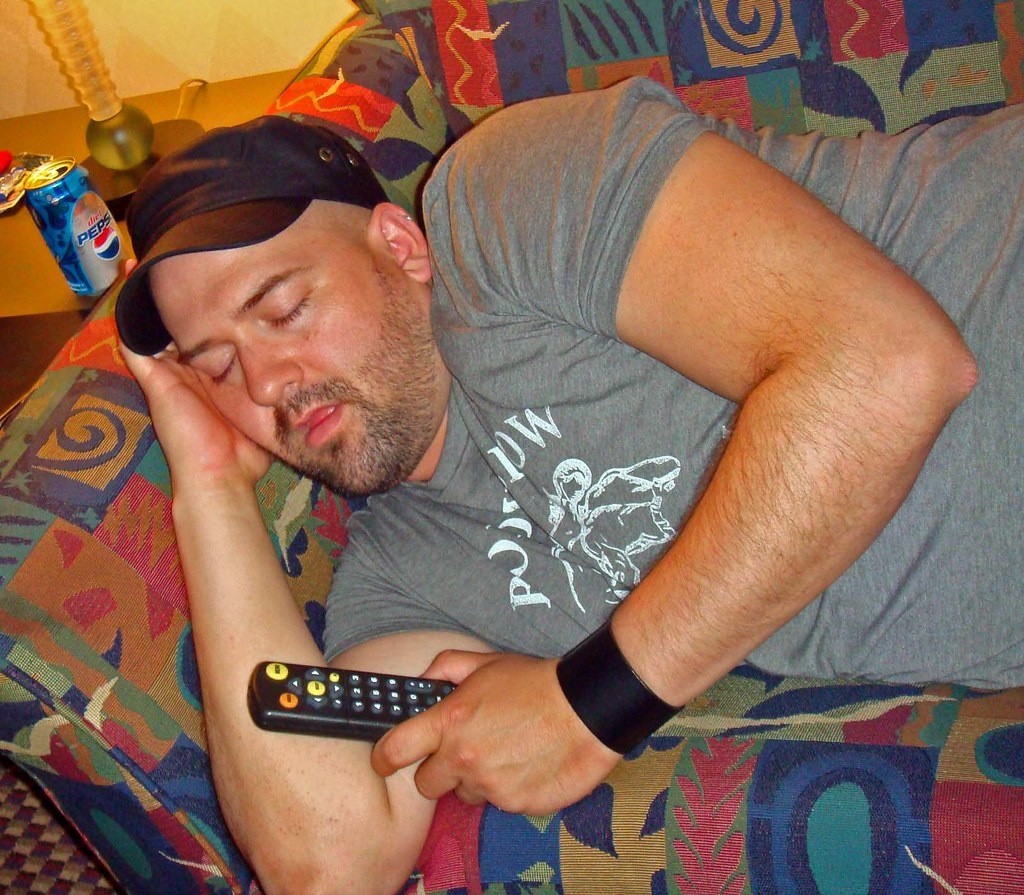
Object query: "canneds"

[22,155,130,296]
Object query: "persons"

[111,78,1024,894]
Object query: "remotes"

[247,662,458,743]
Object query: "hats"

[116,115,393,358]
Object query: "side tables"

[0,69,295,434]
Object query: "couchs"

[0,0,1024,895]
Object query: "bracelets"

[553,619,684,756]
